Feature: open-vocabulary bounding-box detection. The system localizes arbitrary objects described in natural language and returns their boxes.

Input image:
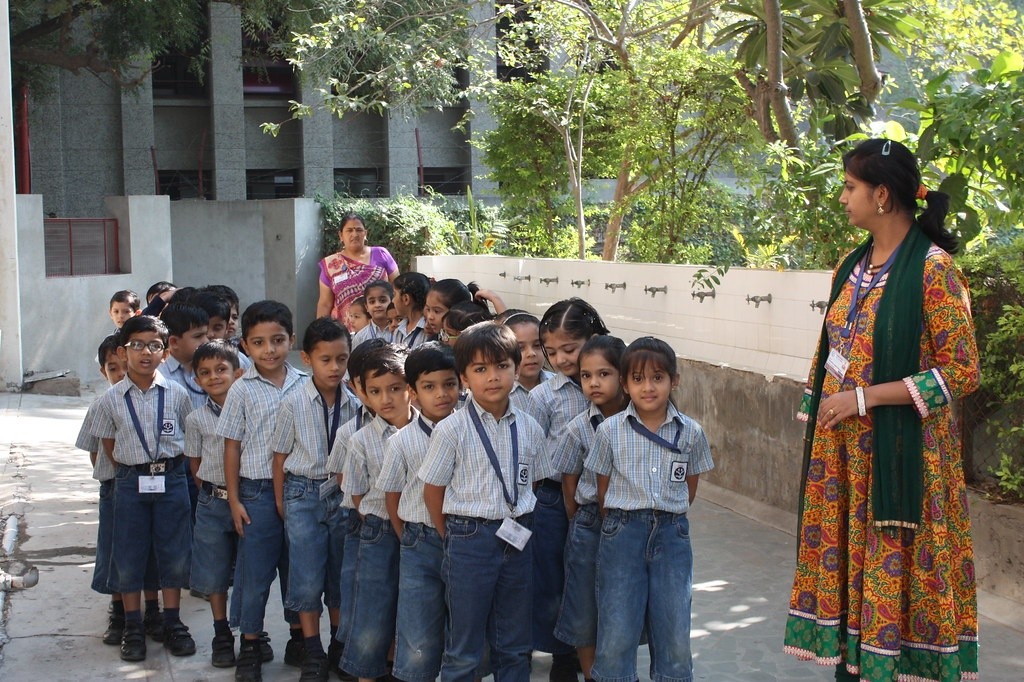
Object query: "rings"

[829,409,835,418]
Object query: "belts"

[468,516,523,524]
[202,480,228,501]
[539,477,561,489]
[140,456,184,473]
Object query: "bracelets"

[856,387,866,417]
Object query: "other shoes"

[189,589,229,601]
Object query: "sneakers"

[234,632,274,682]
[284,638,437,682]
[212,631,236,668]
[120,616,147,662]
[162,619,196,656]
[104,603,162,644]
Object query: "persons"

[786,138,980,682]
[77,272,715,682]
[316,215,400,333]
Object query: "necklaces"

[865,242,878,275]
[869,262,888,269]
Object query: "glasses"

[440,328,458,342]
[124,339,165,353]
